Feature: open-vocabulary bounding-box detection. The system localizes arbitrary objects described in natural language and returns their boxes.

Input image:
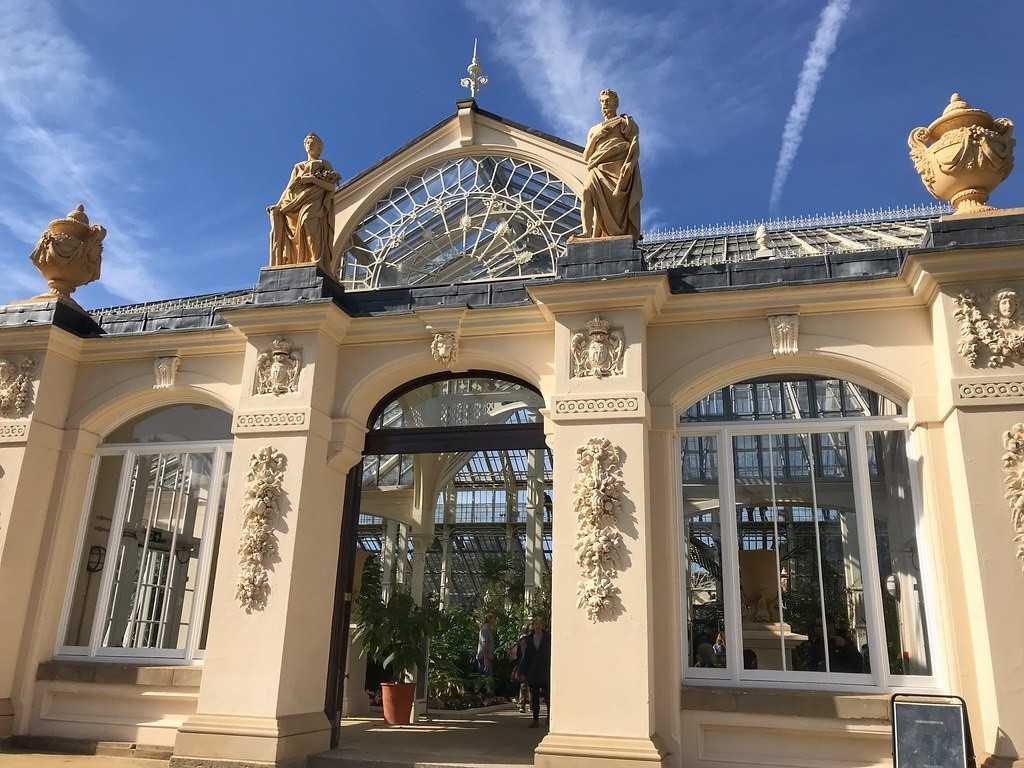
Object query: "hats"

[695,642,716,663]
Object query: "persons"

[519,675,532,713]
[713,633,726,668]
[476,613,495,696]
[573,88,643,238]
[266,133,342,267]
[809,617,864,673]
[518,617,551,728]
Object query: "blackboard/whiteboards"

[890,692,977,768]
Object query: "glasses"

[815,623,828,628]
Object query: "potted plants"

[346,590,446,723]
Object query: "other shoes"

[529,719,539,727]
[545,720,549,729]
[529,705,533,711]
[519,709,526,714]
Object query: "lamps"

[87,546,106,571]
[885,572,902,602]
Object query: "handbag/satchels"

[510,669,520,683]
[507,642,518,661]
